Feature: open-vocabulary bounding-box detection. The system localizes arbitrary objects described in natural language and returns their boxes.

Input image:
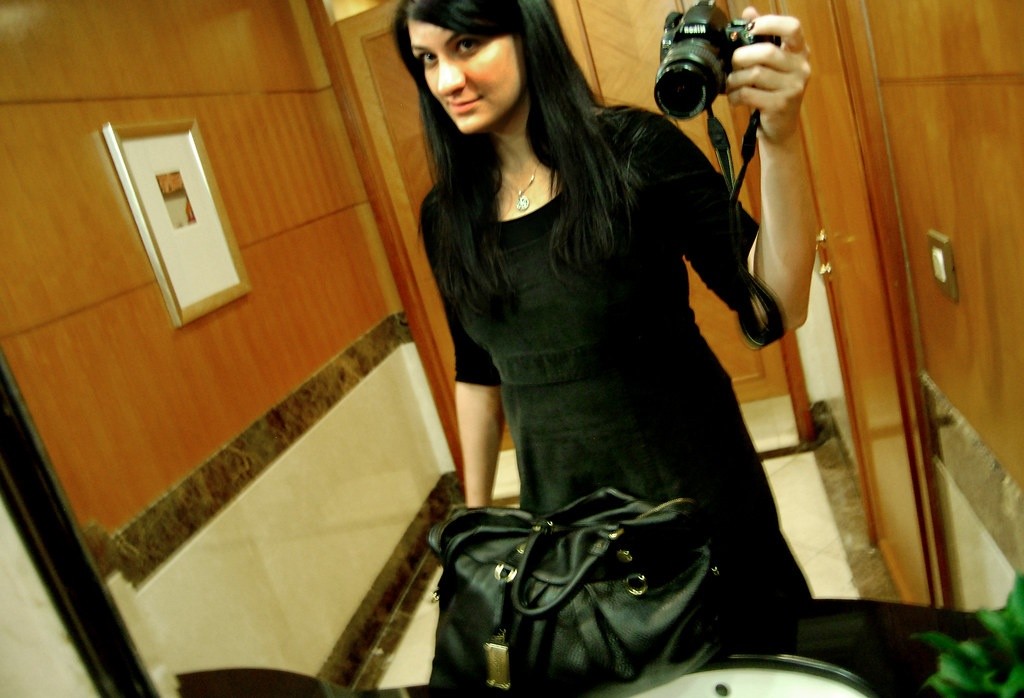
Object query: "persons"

[391,0,818,604]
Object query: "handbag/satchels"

[429,488,720,698]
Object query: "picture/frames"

[99,117,253,329]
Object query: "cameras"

[653,0,776,120]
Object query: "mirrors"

[1,1,1024,698]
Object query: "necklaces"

[502,156,538,212]
